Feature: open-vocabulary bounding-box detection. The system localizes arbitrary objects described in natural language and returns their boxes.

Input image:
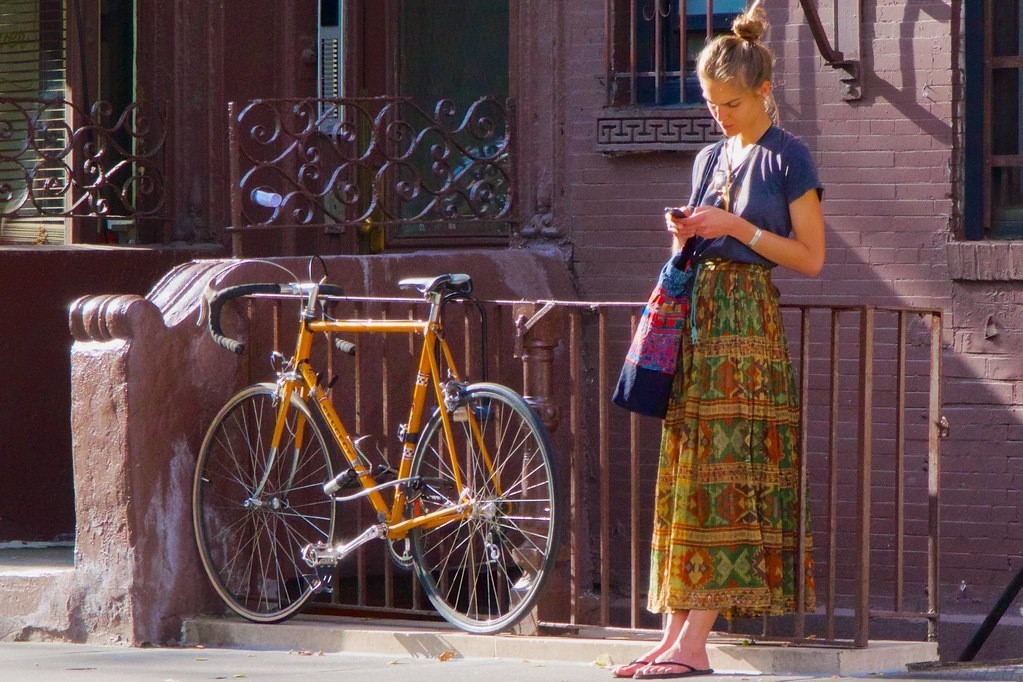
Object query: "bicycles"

[191,253,563,634]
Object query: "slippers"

[633,660,714,679]
[615,659,648,677]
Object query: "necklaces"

[723,135,757,211]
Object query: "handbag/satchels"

[613,242,693,419]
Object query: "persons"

[616,5,825,676]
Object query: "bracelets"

[747,228,763,249]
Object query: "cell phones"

[665,207,688,219]
